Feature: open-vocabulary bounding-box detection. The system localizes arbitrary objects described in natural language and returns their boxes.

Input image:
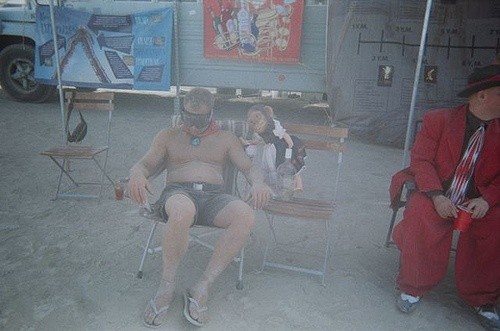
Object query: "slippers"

[183,288,204,326]
[143,292,176,328]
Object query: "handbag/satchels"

[65,91,88,143]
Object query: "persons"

[126,88,270,328]
[395,64,500,331]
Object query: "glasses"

[181,113,209,129]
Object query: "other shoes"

[472,306,500,331]
[397,292,419,314]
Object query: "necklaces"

[191,137,200,146]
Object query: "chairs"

[254,120,346,286]
[136,114,248,289]
[41,91,116,204]
[385,119,455,254]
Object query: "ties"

[445,123,486,207]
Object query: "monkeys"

[246,104,275,135]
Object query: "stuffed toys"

[241,102,306,192]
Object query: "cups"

[114,183,124,200]
[452,205,474,231]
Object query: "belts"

[178,181,218,191]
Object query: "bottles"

[275,149,297,203]
[206,0,258,48]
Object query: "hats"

[457,64,500,98]
